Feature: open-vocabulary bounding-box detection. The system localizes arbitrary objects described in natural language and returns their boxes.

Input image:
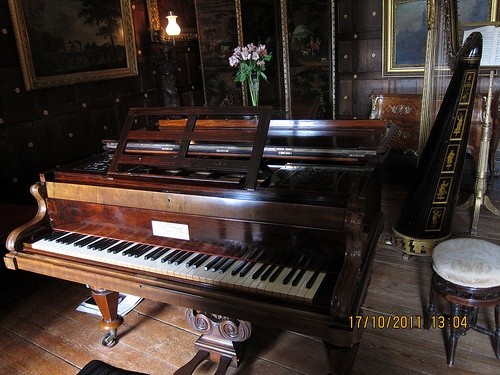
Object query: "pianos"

[3,106,403,375]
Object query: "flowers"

[227,42,273,105]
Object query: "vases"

[247,73,261,118]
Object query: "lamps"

[154,10,181,48]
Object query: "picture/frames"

[7,0,139,93]
[382,0,500,79]
[146,0,197,42]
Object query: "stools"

[422,238,500,365]
